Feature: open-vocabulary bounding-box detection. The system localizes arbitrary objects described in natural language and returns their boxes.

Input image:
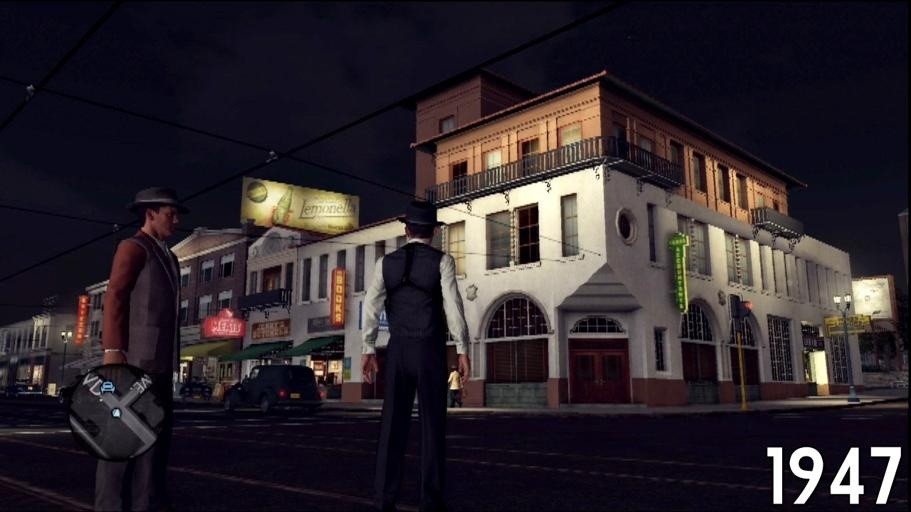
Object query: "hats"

[397,199,445,227]
[126,188,191,216]
